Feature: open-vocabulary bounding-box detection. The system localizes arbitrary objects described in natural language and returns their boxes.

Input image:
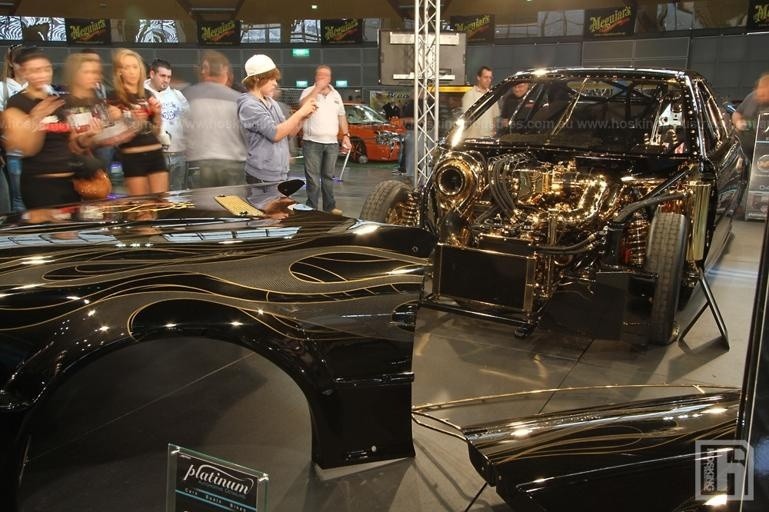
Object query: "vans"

[403,86,472,135]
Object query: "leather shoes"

[330,208,344,216]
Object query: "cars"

[285,100,407,162]
[359,66,753,343]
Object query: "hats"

[242,54,277,85]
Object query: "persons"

[462,67,500,145]
[732,74,769,161]
[3,46,352,216]
[381,94,402,119]
[502,73,538,127]
